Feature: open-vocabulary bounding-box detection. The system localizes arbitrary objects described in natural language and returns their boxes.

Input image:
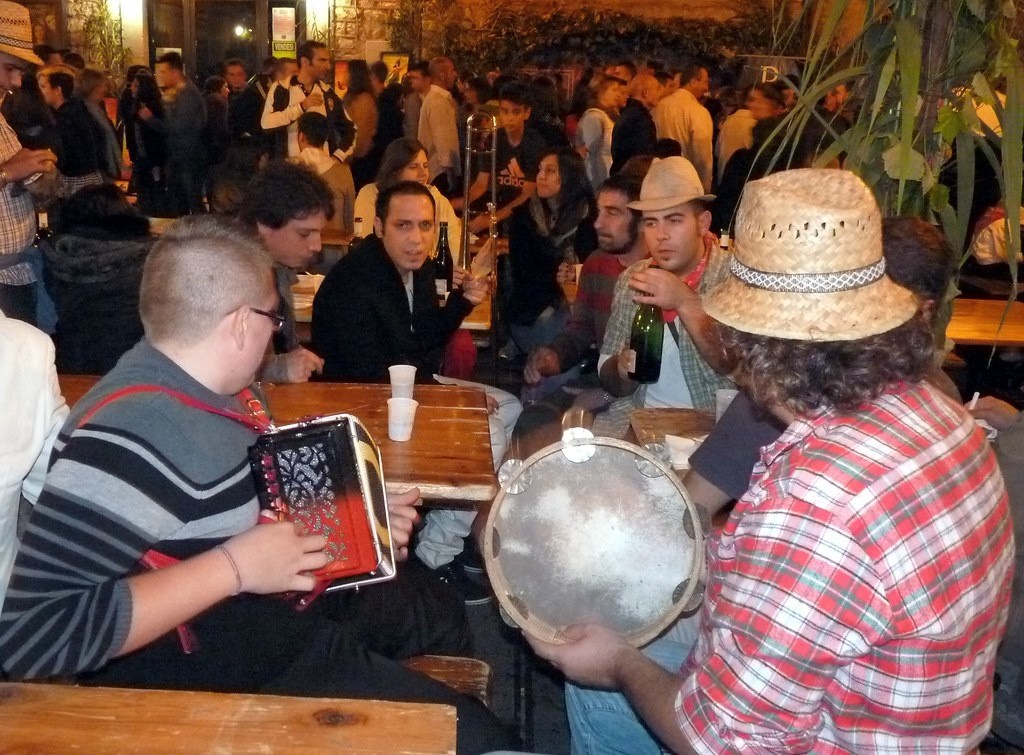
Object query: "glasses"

[227,308,282,330]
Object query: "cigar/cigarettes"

[968,392,980,410]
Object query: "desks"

[0,277,1024,755]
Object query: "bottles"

[719,230,730,251]
[627,261,663,385]
[431,220,453,301]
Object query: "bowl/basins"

[289,274,325,312]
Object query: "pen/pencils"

[968,392,980,410]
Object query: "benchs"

[389,655,494,710]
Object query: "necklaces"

[651,230,713,324]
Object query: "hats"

[701,168,919,342]
[626,156,717,211]
[0,1,45,65]
[36,63,77,80]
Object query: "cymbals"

[477,426,712,651]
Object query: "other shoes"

[498,338,518,360]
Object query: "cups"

[387,398,419,442]
[715,390,742,423]
[574,264,583,285]
[388,365,417,400]
[253,382,277,414]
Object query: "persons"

[0,39,1006,233]
[964,395,1024,755]
[253,158,335,384]
[310,179,494,607]
[969,196,1023,302]
[523,166,1015,755]
[27,4,57,45]
[33,181,158,377]
[577,156,743,440]
[502,146,598,381]
[519,172,654,408]
[682,215,960,518]
[0,309,70,619]
[353,138,479,266]
[1,1,57,337]
[0,218,519,754]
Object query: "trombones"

[456,111,497,297]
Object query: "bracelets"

[0,170,8,191]
[216,545,241,596]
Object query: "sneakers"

[431,561,493,604]
[455,552,485,573]
